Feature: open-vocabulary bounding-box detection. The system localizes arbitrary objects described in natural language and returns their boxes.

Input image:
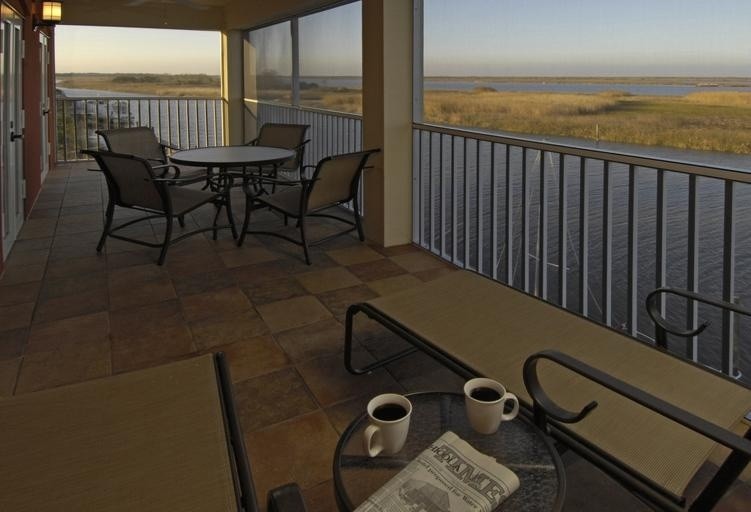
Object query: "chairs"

[217,124,311,193]
[346,267,751,511]
[0,352,307,512]
[80,149,224,265]
[236,149,381,266]
[95,127,211,191]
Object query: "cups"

[463,377,519,435]
[362,393,412,458]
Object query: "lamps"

[33,0,64,32]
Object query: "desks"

[169,146,296,243]
